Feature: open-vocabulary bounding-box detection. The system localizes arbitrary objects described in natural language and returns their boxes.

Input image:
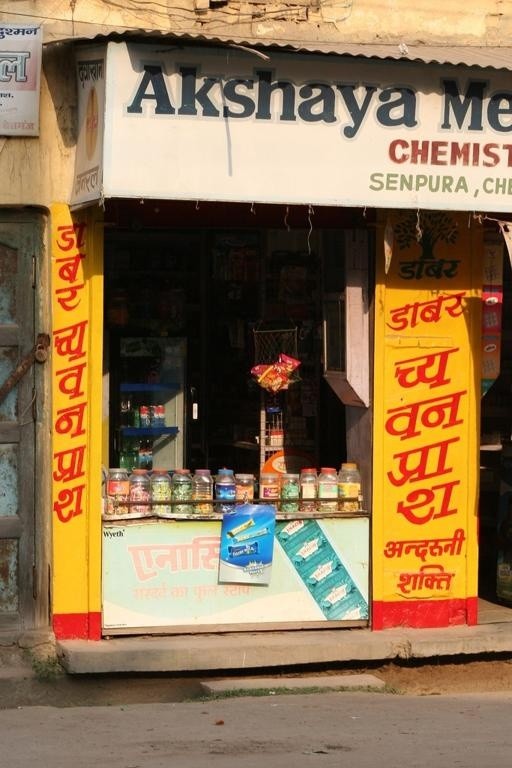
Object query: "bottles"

[299,468,319,513]
[318,467,339,512]
[192,469,215,514]
[338,463,364,512]
[128,468,152,514]
[280,473,301,512]
[171,468,193,514]
[235,473,254,505]
[106,468,130,515]
[149,467,172,514]
[115,399,166,469]
[258,473,279,512]
[214,469,236,514]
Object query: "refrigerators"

[109,327,190,470]
